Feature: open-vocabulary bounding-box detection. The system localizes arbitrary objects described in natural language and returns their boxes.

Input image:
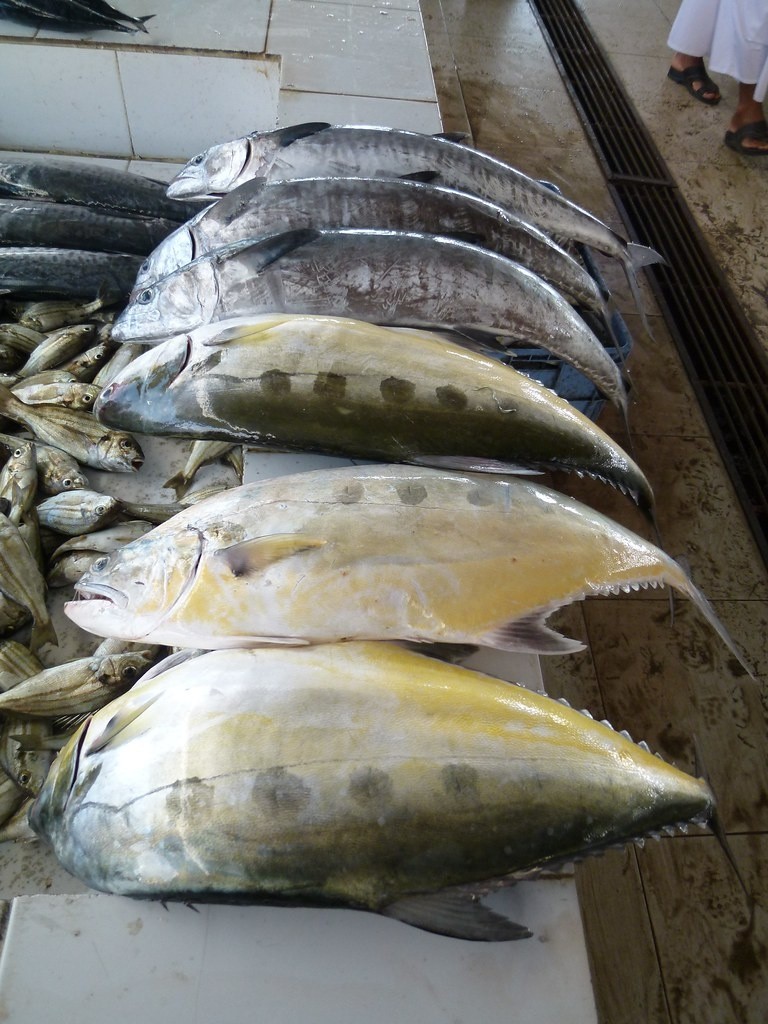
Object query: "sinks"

[0,37,287,995]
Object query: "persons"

[663,1,768,156]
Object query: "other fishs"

[0,118,768,942]
[0,0,158,37]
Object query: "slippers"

[725,120,768,156]
[668,66,722,105]
